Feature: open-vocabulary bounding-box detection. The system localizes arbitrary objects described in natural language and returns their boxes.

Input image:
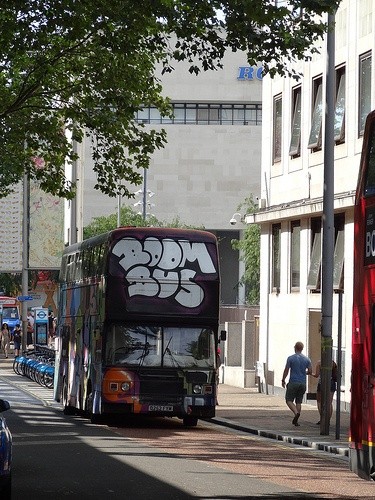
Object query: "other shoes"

[291,413,300,425]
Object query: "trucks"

[0,295,20,342]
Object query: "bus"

[52,228,227,427]
[348,110,375,482]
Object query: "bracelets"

[282,379,285,384]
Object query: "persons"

[0,322,11,359]
[26,310,57,347]
[215,347,223,406]
[311,358,342,427]
[13,324,23,359]
[281,341,312,427]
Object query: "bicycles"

[12,343,56,389]
